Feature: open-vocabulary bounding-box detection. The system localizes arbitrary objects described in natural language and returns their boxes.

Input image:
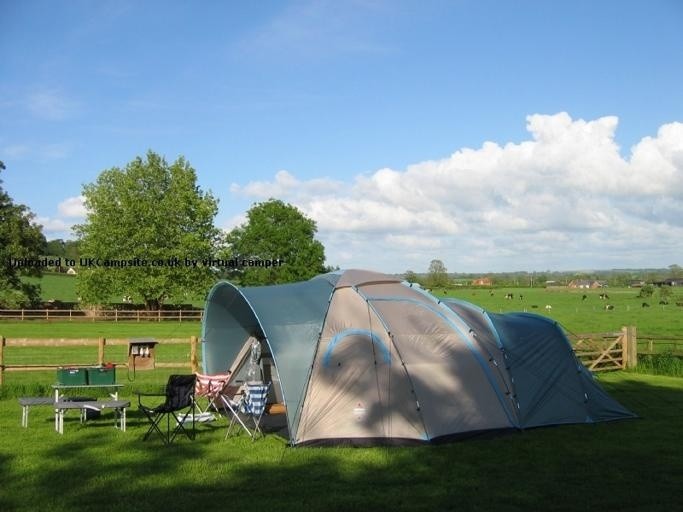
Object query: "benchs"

[19,397,97,428]
[52,384,125,403]
[52,402,130,435]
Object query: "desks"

[132,383,168,444]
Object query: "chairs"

[189,336,257,422]
[137,374,197,449]
[218,381,273,442]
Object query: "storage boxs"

[57,367,88,386]
[88,366,116,385]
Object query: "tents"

[201,267,643,449]
